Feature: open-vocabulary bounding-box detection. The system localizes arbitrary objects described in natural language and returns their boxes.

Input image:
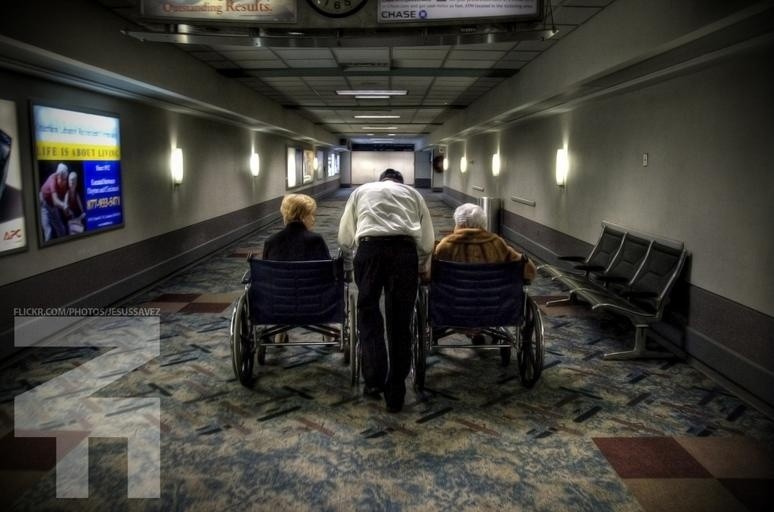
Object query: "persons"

[338,169,435,412]
[435,202,537,360]
[64,171,85,219]
[262,193,337,348]
[39,163,73,243]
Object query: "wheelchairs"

[227,246,362,388]
[410,253,545,397]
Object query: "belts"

[360,236,413,242]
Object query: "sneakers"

[363,384,386,396]
[385,393,405,413]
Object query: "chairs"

[535,219,688,360]
